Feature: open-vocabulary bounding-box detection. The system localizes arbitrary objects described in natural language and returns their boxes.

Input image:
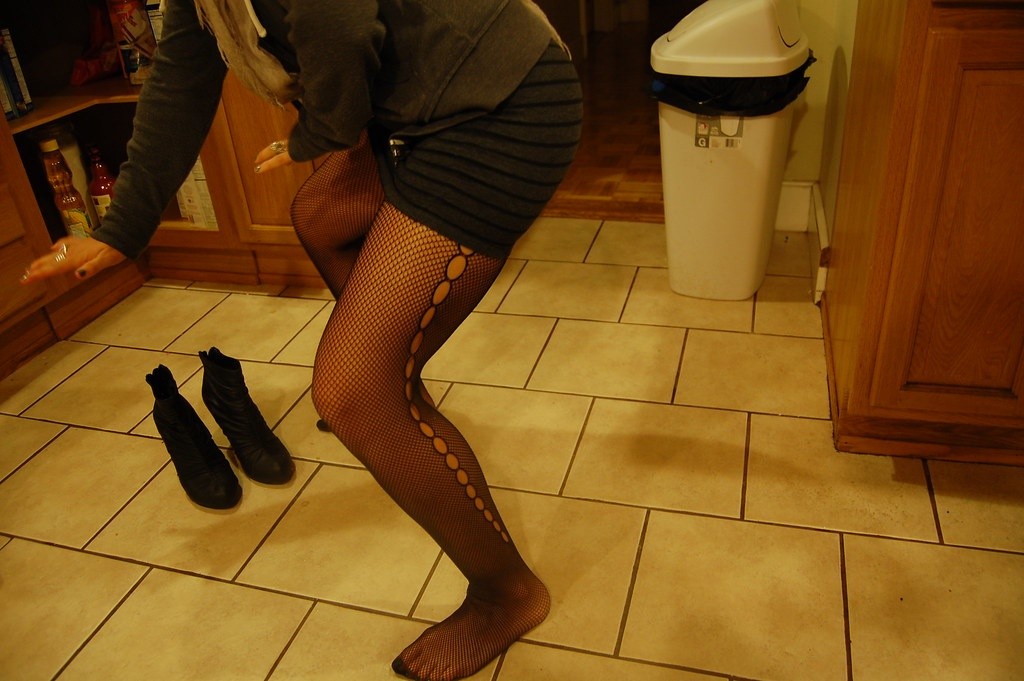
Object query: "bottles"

[107,0,156,85]
[90,148,115,227]
[45,122,100,230]
[39,139,91,239]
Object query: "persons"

[21,0,583,681]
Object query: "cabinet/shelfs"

[820,1,1024,465]
[0,0,342,377]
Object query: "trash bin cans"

[641,0,817,302]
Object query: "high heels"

[198,347,296,485]
[145,364,242,509]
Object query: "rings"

[270,140,287,153]
[59,243,67,254]
[55,252,65,262]
